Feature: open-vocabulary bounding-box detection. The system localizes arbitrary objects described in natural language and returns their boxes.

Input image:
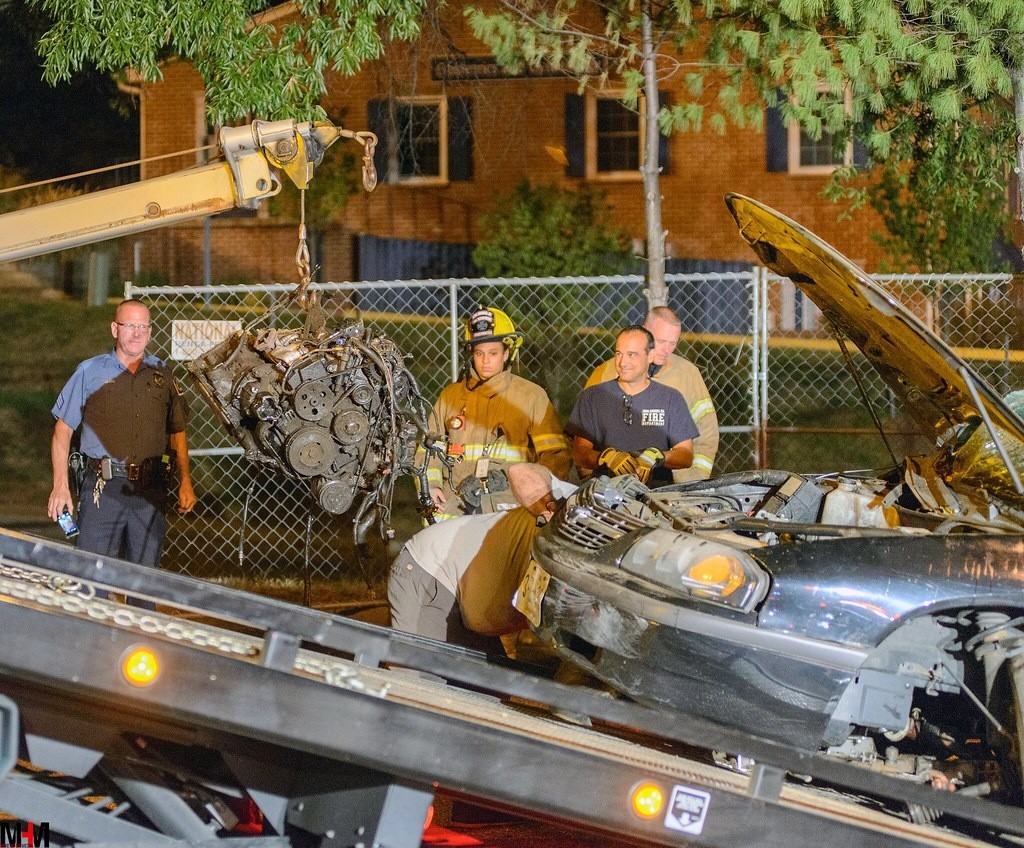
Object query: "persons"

[382,461,580,653]
[560,324,700,487]
[580,306,721,483]
[410,306,573,525]
[47,300,196,608]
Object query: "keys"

[92,471,106,508]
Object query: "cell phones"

[57,509,80,540]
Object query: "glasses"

[115,321,150,331]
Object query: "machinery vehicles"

[2,109,1024,848]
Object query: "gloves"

[633,447,664,488]
[598,446,639,479]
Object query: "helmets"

[465,304,519,345]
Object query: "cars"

[495,192,1022,805]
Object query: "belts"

[84,456,142,480]
